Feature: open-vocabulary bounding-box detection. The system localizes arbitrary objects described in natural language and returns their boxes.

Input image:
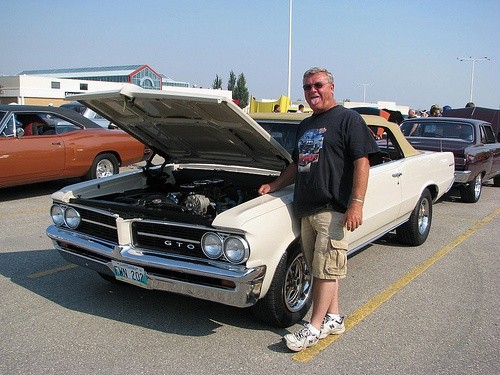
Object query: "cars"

[46,86,455,324]
[0,105,146,188]
[59,101,121,128]
[400,106,500,203]
[349,107,390,139]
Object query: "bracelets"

[351,197,364,203]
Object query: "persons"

[296,104,305,113]
[273,104,280,113]
[404,101,475,136]
[257,67,371,354]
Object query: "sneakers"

[282,323,318,351]
[319,314,345,339]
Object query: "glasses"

[302,82,329,90]
